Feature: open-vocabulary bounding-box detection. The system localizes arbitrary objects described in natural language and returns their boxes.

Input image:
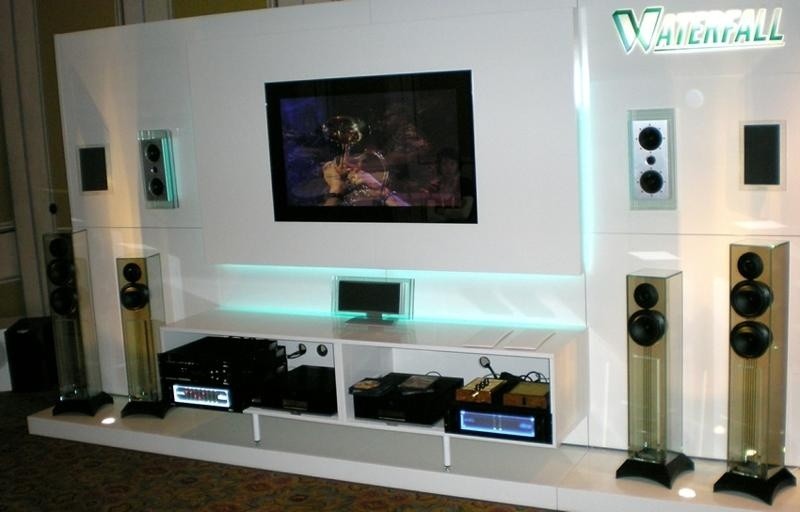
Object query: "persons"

[321,102,460,206]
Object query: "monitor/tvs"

[264,71,478,223]
[730,239,789,480]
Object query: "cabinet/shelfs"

[158,311,590,472]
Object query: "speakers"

[139,138,171,201]
[625,267,684,465]
[43,227,101,400]
[631,120,669,203]
[117,251,165,401]
